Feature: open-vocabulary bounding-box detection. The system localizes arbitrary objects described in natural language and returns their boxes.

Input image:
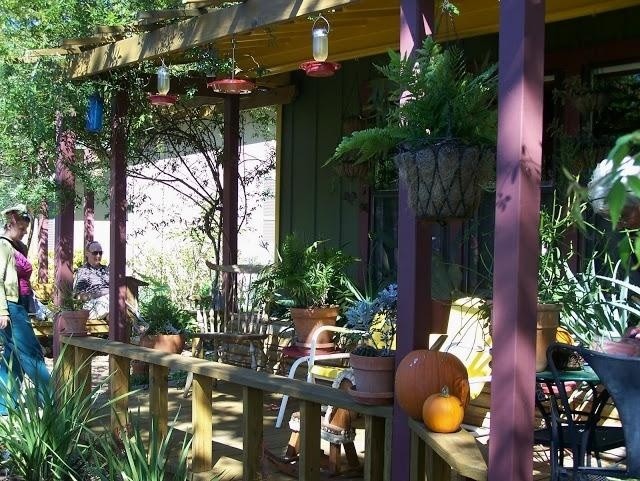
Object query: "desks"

[536,364,609,481]
[282,346,343,375]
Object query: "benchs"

[30,283,109,337]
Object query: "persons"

[1,205,55,417]
[74,241,150,336]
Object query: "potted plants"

[48,279,105,335]
[241,226,362,344]
[321,2,499,218]
[345,284,398,394]
[484,224,576,373]
[131,281,198,354]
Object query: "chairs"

[183,260,275,398]
[275,296,492,428]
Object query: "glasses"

[91,250,102,255]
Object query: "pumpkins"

[422,384,463,434]
[394,333,470,423]
